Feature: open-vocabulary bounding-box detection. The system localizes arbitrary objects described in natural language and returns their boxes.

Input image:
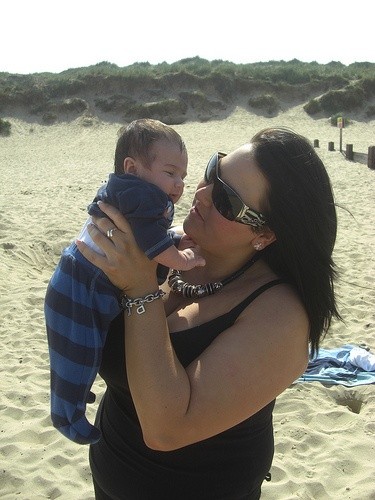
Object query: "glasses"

[204,151,268,229]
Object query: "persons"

[89,125,361,500]
[44,118,205,445]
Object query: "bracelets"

[122,289,166,315]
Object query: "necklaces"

[168,250,261,298]
